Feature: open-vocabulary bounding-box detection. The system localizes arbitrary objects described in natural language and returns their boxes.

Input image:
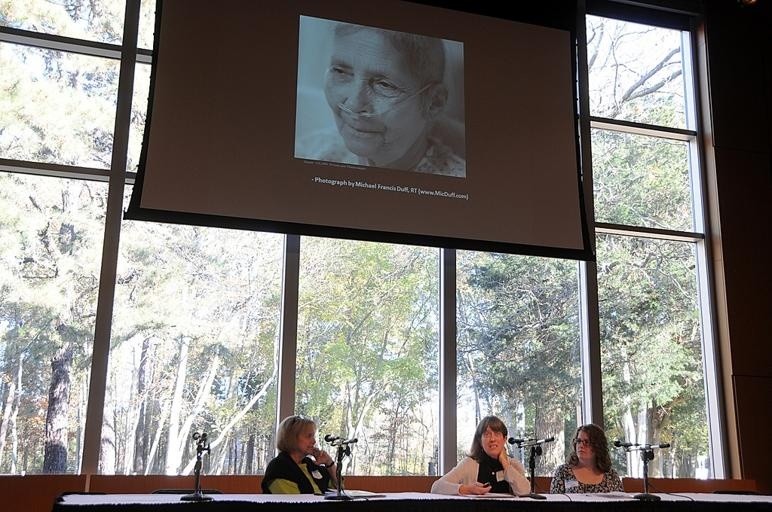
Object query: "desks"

[51,490,771,511]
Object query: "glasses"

[573,438,592,447]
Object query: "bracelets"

[325,461,334,468]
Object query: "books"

[325,489,385,500]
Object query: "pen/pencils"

[364,497,369,500]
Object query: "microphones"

[324,435,339,442]
[193,431,201,440]
[614,441,640,447]
[508,438,533,445]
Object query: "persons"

[432,416,531,496]
[261,415,345,494]
[294,22,466,178]
[550,424,624,494]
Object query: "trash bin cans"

[428,462,434,474]
